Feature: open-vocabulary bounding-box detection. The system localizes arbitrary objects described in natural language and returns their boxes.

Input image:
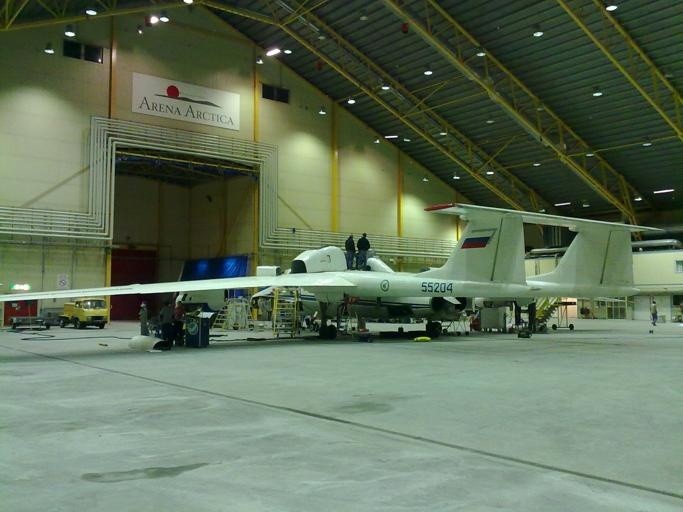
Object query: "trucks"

[53,295,111,330]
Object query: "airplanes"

[0,197,683,342]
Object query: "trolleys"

[550,301,575,331]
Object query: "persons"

[345,234,356,270]
[357,233,370,270]
[160,299,185,340]
[650,301,658,326]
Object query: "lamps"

[533,23,543,38]
[438,126,448,136]
[585,147,595,157]
[318,106,327,116]
[319,28,325,41]
[605,1,618,11]
[476,48,487,58]
[382,82,390,91]
[359,9,368,21]
[592,86,603,97]
[423,65,433,75]
[487,114,494,124]
